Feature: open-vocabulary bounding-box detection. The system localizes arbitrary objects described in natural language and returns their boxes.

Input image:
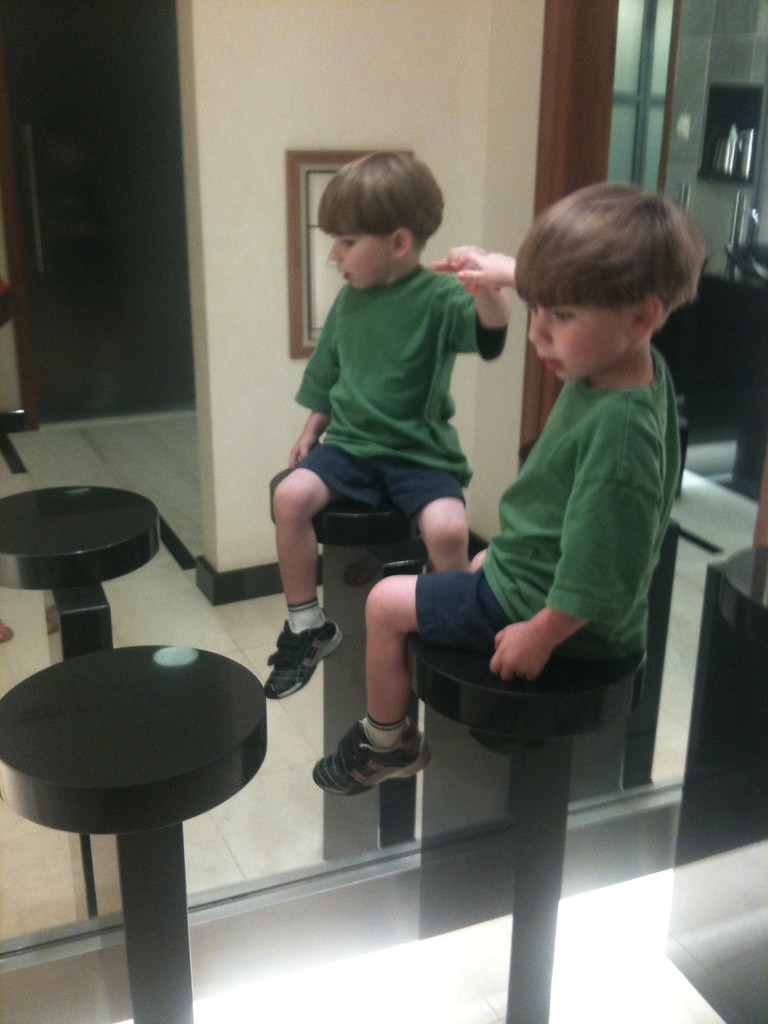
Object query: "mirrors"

[0,0,768,1024]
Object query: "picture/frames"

[281,147,412,357]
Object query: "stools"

[0,486,160,918]
[267,470,422,860]
[0,642,270,1024]
[416,647,643,1024]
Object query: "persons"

[264,151,511,699]
[312,182,702,795]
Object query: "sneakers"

[264,609,345,699]
[313,719,432,796]
